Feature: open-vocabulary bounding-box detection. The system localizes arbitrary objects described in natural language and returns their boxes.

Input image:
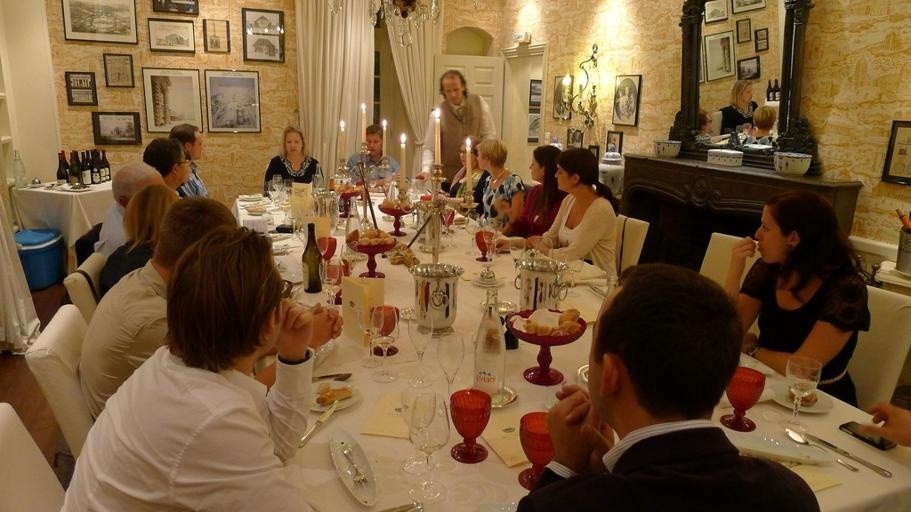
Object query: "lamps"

[326,0,479,51]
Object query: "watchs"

[748,344,761,356]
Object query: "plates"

[27,181,92,191]
[469,385,516,409]
[772,384,834,414]
[309,379,359,412]
[328,424,378,508]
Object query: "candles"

[340,103,472,191]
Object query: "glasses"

[270,279,293,309]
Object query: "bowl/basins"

[773,151,812,177]
[655,139,681,160]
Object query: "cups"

[449,389,493,467]
[480,300,521,350]
[513,258,569,309]
[409,263,464,338]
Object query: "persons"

[79,197,343,417]
[99,124,210,297]
[699,109,714,132]
[493,148,620,274]
[709,82,758,137]
[615,80,637,120]
[450,134,570,237]
[345,126,402,187]
[863,401,911,448]
[726,189,867,409]
[265,125,323,192]
[413,69,493,192]
[753,105,777,140]
[516,265,822,510]
[59,223,316,512]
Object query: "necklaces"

[493,167,508,183]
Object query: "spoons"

[786,427,858,472]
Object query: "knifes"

[805,432,892,476]
[297,400,339,449]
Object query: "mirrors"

[669,0,822,177]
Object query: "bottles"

[472,289,506,405]
[13,150,28,188]
[607,275,619,294]
[56,149,111,186]
[767,78,781,102]
[302,156,399,294]
[895,208,911,234]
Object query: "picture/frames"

[704,0,728,25]
[881,120,911,185]
[527,75,641,160]
[732,0,766,14]
[737,55,760,80]
[704,30,736,82]
[699,36,706,85]
[736,18,751,43]
[62,0,285,145]
[755,28,769,53]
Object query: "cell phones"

[839,421,897,451]
[276,224,293,233]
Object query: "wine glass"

[437,333,466,417]
[398,386,436,474]
[268,174,588,288]
[407,309,433,388]
[357,305,385,368]
[408,392,452,505]
[779,356,823,433]
[720,366,766,431]
[318,260,344,308]
[369,306,399,386]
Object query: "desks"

[621,153,864,237]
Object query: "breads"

[316,381,331,395]
[346,226,394,245]
[788,382,819,408]
[523,308,583,337]
[316,386,355,406]
